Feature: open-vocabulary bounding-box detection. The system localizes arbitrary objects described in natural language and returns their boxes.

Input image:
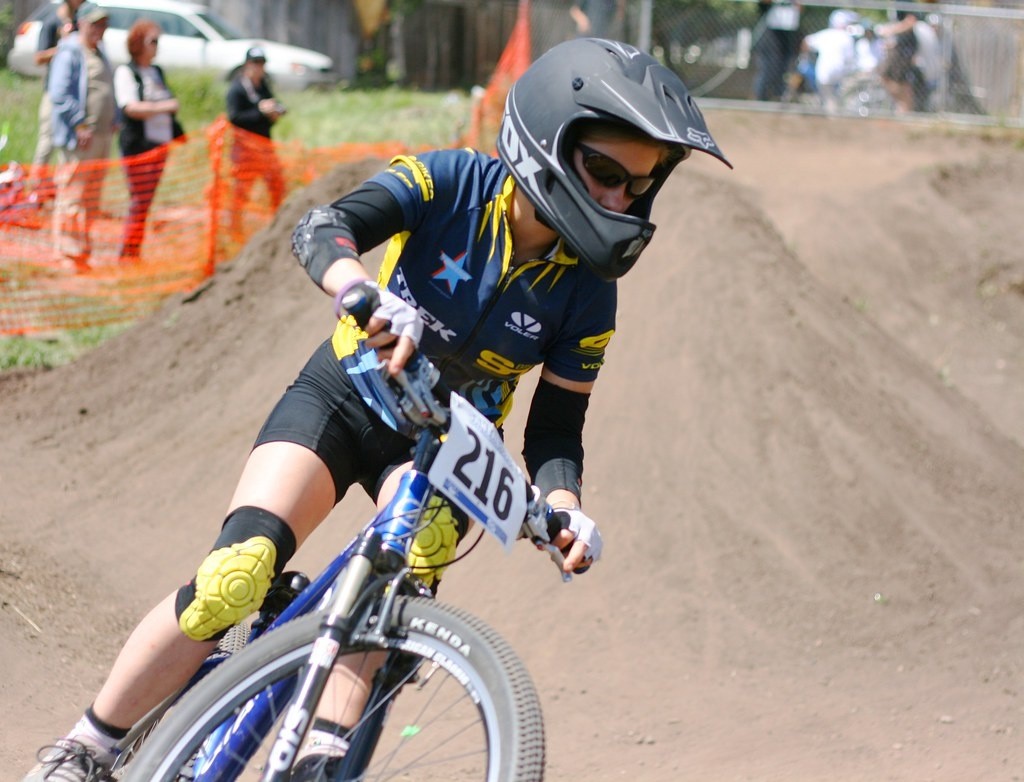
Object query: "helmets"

[495,38,732,282]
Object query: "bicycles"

[84,283,591,782]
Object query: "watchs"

[550,499,582,511]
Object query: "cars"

[6,1,341,99]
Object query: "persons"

[751,0,983,115]
[111,20,187,262]
[564,0,625,41]
[26,0,114,274]
[224,45,286,244]
[7,36,733,782]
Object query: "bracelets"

[334,277,373,328]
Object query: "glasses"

[143,40,158,44]
[576,142,655,200]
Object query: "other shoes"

[288,729,350,782]
[25,731,117,782]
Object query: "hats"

[76,0,109,23]
[248,48,268,64]
